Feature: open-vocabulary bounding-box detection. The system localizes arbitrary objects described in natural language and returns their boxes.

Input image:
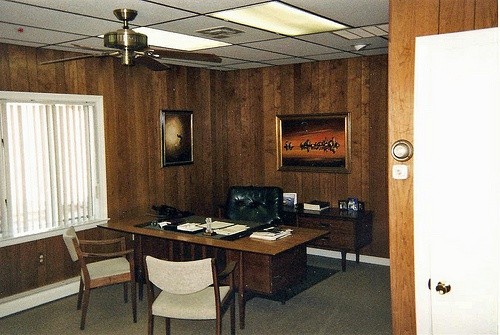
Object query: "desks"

[97,214,331,329]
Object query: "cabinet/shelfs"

[283,204,374,271]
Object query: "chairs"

[63,226,138,330]
[143,255,236,335]
[226,186,283,227]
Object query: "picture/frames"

[276,111,350,174]
[160,109,194,168]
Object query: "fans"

[37,8,222,71]
